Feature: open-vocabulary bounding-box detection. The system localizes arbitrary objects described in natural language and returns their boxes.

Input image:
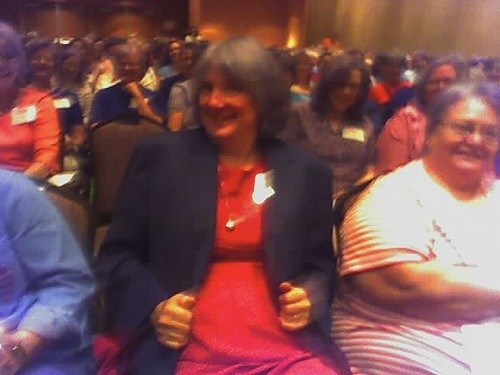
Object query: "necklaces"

[216,161,261,229]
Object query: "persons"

[0,17,500,196]
[329,76,500,374]
[99,36,353,375]
[0,166,102,375]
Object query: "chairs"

[91,116,166,255]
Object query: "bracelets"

[2,338,29,366]
